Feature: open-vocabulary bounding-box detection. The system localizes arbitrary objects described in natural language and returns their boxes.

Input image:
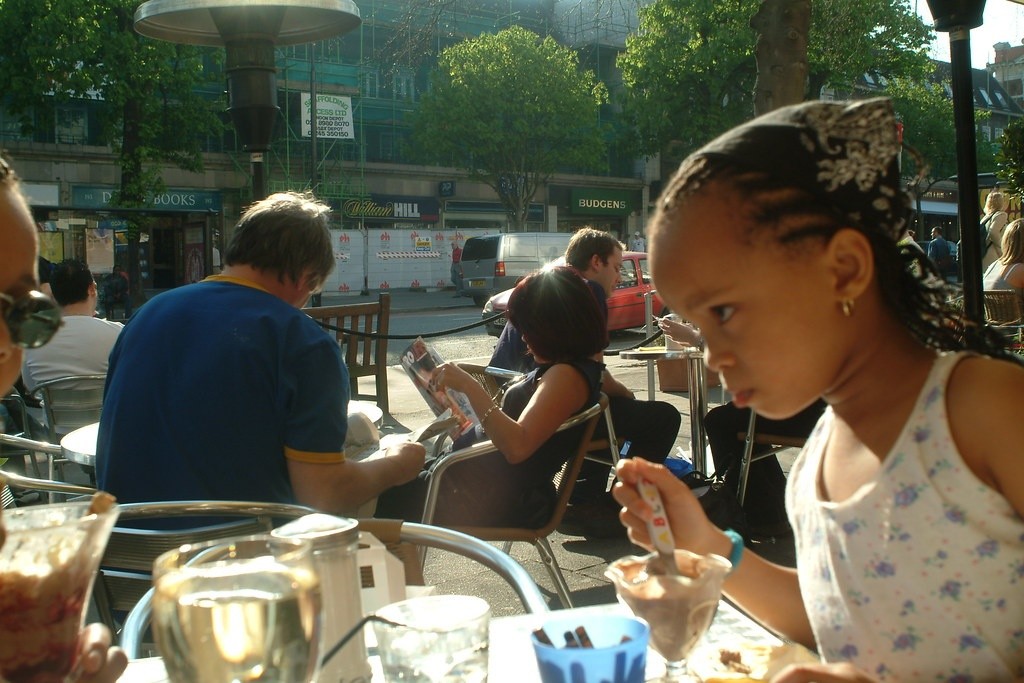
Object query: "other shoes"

[749,519,790,537]
[556,497,622,536]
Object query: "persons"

[612,96,1024,683]
[20,256,127,431]
[10,303,38,344]
[897,189,1024,348]
[450,242,462,298]
[631,231,647,253]
[490,228,681,535]
[96,191,428,645]
[376,267,606,528]
[660,319,827,540]
[0,165,129,683]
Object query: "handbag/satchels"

[978,214,1003,257]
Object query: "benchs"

[301,293,389,412]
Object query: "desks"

[58,399,382,488]
[618,347,709,479]
[113,590,814,683]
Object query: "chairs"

[0,284,1024,662]
[618,264,630,285]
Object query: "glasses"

[0,290,65,349]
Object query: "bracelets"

[481,404,499,428]
[721,529,743,580]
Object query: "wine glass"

[602,549,732,683]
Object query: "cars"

[482,251,672,342]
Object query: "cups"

[150,536,321,682]
[533,610,650,683]
[1,502,121,683]
[372,594,489,682]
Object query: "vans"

[459,232,578,306]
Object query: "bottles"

[662,313,684,351]
[270,512,370,682]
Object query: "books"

[399,336,480,440]
[345,409,462,467]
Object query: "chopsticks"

[533,626,631,648]
